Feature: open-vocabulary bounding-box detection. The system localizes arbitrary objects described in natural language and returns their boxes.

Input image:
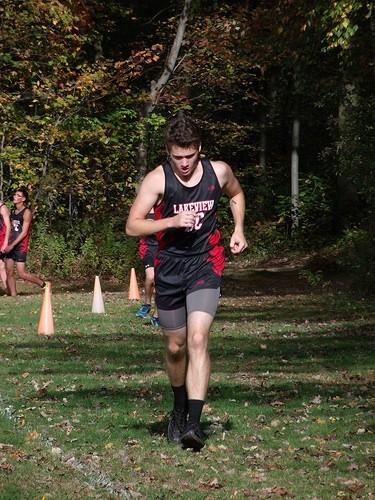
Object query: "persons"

[125,117,248,452]
[4,190,50,296]
[0,192,11,297]
[139,208,159,327]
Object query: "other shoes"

[42,282,48,291]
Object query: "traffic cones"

[128,267,141,301]
[37,281,57,337]
[91,275,106,314]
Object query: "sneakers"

[151,314,160,327]
[167,410,205,448]
[136,304,151,317]
